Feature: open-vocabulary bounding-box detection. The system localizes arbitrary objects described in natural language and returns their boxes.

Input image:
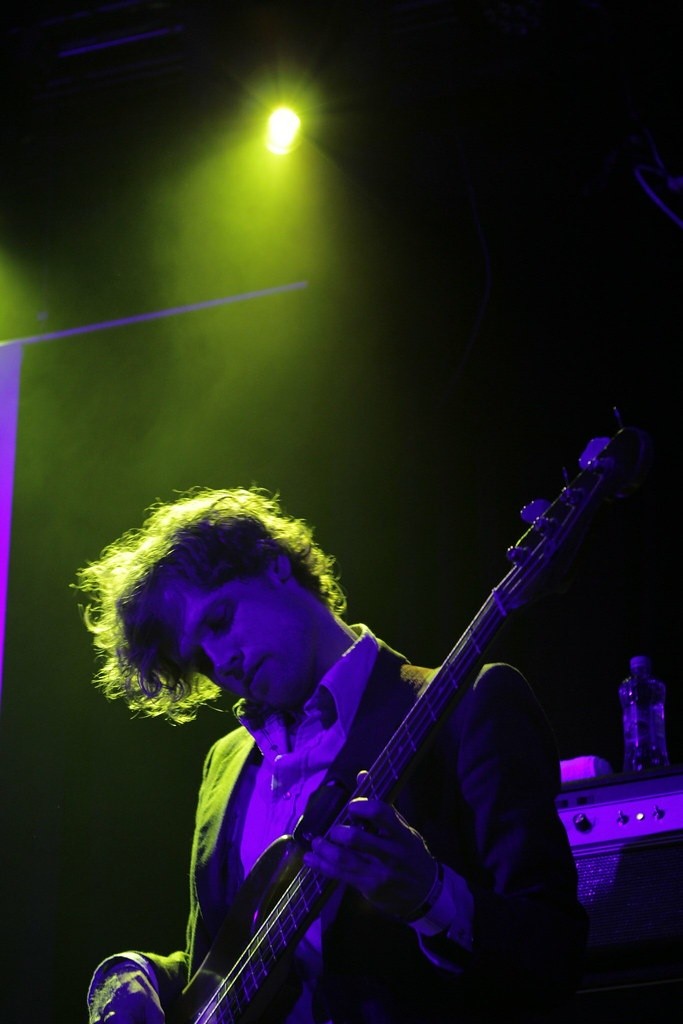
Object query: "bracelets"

[401,860,444,921]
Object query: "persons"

[77,488,579,1024]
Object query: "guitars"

[156,405,651,1023]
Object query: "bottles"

[619,657,670,775]
